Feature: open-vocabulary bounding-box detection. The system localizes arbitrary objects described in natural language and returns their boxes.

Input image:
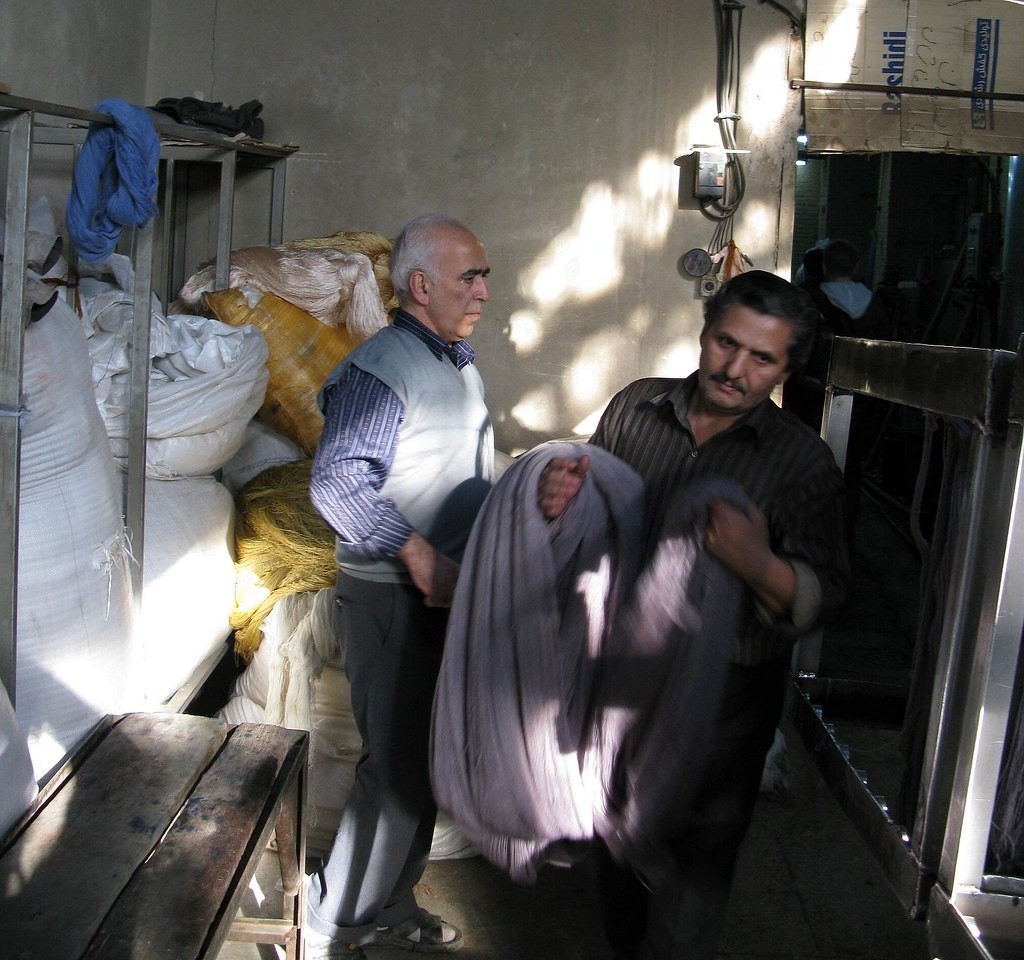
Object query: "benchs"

[0,711,310,959]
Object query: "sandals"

[372,909,464,954]
[309,934,367,960]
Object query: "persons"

[537,269,848,960]
[309,214,511,959]
